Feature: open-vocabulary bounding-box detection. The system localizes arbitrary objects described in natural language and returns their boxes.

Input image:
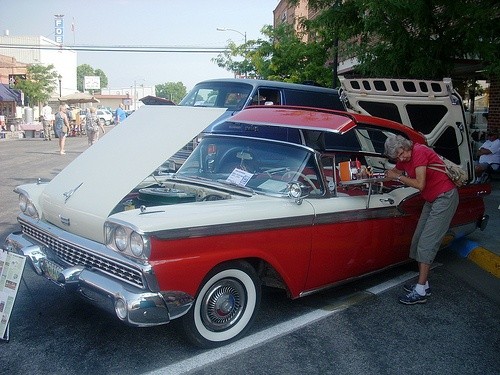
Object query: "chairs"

[479,163,500,184]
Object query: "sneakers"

[398,289,428,304]
[400,280,431,296]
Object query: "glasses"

[391,148,408,161]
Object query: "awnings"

[0,83,21,102]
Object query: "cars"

[1,76,490,350]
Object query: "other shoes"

[59,149,66,152]
[59,151,66,155]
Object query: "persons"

[41,100,127,155]
[382,134,460,305]
[470,129,500,184]
[255,93,276,105]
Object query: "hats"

[485,129,500,137]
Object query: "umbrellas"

[58,92,101,136]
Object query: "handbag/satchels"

[415,143,469,189]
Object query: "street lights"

[216,27,247,78]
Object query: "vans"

[176,77,348,113]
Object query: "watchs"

[396,174,402,182]
